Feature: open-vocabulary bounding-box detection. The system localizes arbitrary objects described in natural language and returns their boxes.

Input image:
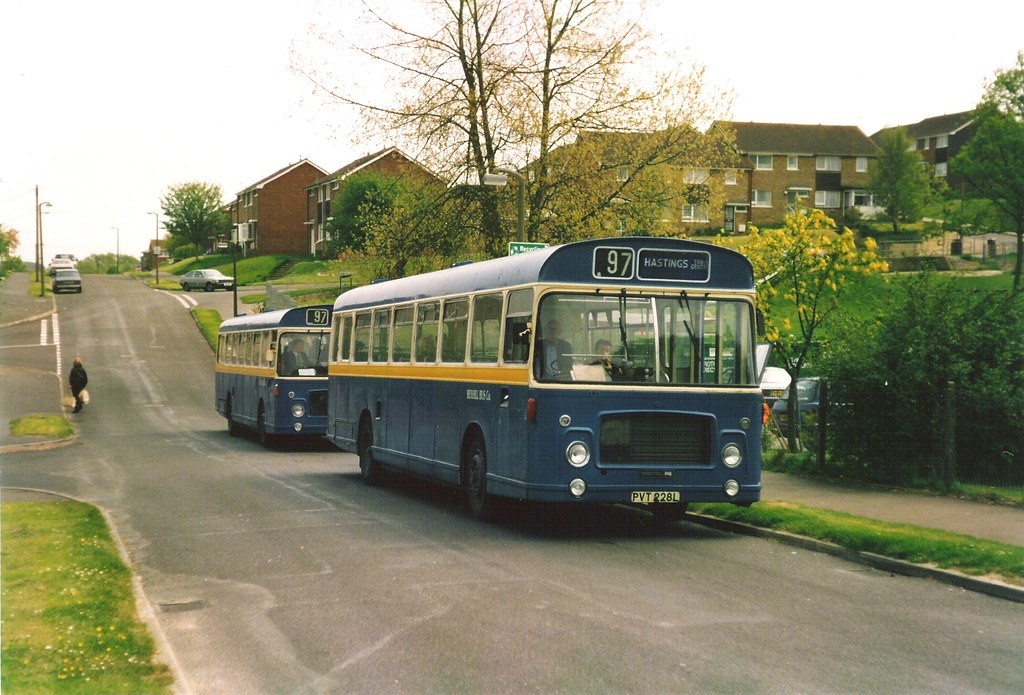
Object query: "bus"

[323,233,765,521]
[213,302,335,447]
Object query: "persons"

[535,320,573,378]
[280,339,316,375]
[585,338,612,364]
[69,357,88,414]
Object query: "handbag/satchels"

[62,388,76,408]
[78,389,89,405]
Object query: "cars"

[51,268,83,293]
[721,366,792,424]
[179,268,234,292]
[48,253,79,274]
[773,376,860,451]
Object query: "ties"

[297,355,302,363]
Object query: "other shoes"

[72,411,79,413]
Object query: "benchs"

[221,355,259,366]
[335,346,498,362]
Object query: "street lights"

[111,226,119,273]
[146,211,159,284]
[38,201,53,298]
